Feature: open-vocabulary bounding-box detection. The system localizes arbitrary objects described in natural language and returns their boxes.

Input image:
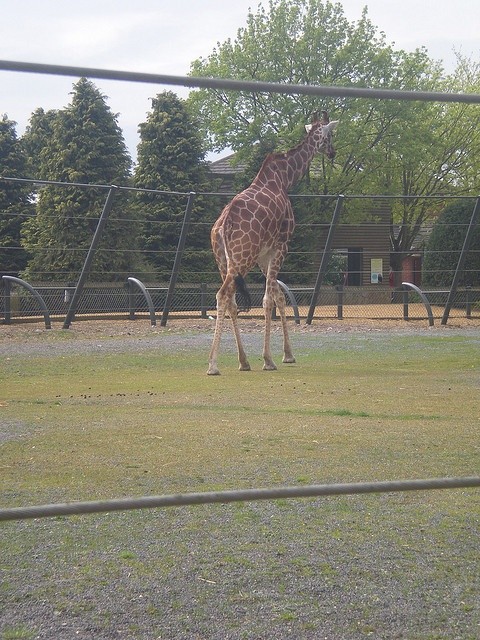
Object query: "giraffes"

[206,109,339,375]
